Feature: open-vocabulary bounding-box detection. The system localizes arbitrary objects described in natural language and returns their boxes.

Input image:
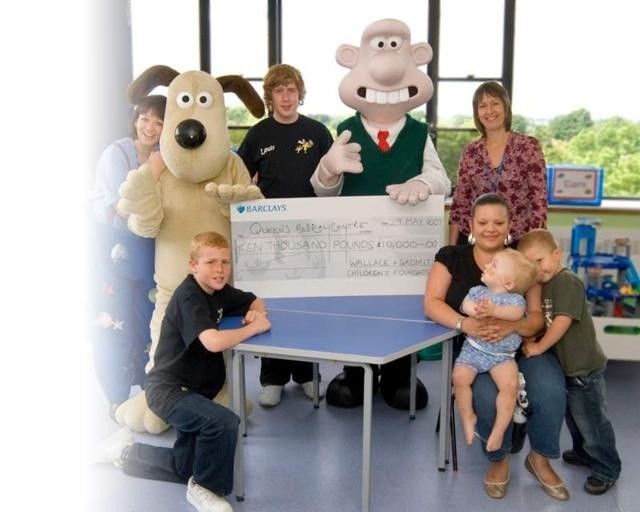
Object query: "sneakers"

[562,448,594,469]
[301,379,326,399]
[583,471,618,494]
[186,475,234,512]
[104,426,134,469]
[257,385,285,408]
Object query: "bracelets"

[456,317,465,334]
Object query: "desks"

[217,295,463,463]
[214,307,465,512]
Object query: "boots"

[510,421,527,454]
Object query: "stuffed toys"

[116,63,266,437]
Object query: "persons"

[423,192,570,503]
[517,229,623,497]
[452,246,539,454]
[235,64,335,410]
[93,231,273,512]
[95,93,166,418]
[309,17,452,413]
[447,80,549,454]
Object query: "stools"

[433,367,461,475]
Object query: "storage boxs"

[545,161,604,209]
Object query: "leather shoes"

[524,452,570,502]
[484,463,510,499]
